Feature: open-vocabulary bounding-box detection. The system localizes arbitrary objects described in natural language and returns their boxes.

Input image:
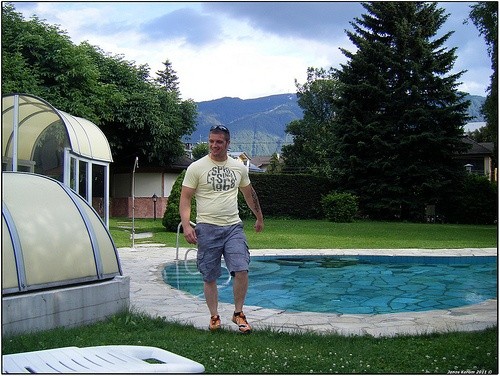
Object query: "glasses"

[210,125,229,133]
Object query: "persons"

[179,125,264,333]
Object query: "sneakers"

[232,311,251,334]
[210,315,221,330]
